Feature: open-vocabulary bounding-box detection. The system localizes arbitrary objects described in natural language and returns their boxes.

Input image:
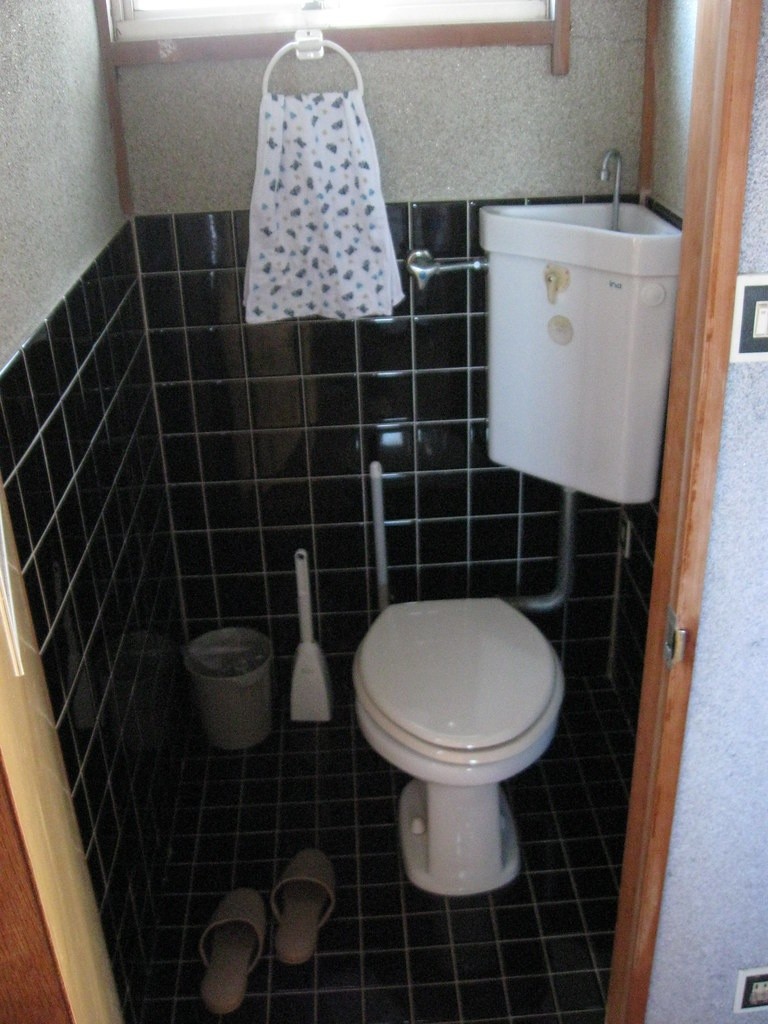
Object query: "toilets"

[350,594,567,900]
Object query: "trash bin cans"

[186,626,274,751]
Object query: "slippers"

[271,849,336,965]
[200,888,266,1015]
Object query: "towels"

[240,86,408,325]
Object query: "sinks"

[478,202,682,277]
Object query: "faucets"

[597,146,624,233]
